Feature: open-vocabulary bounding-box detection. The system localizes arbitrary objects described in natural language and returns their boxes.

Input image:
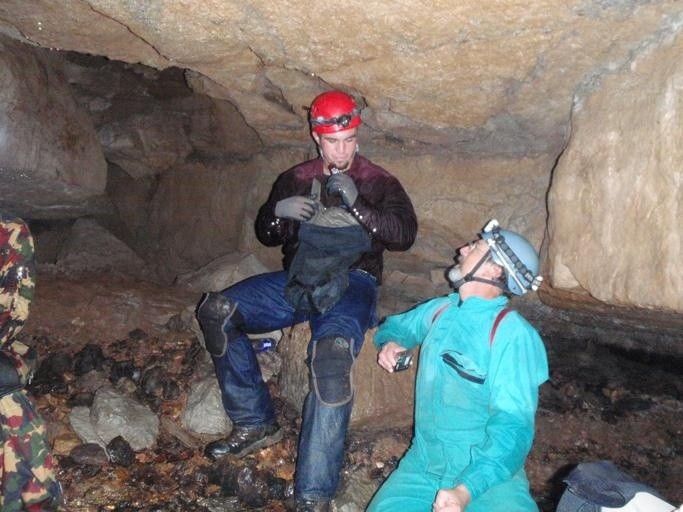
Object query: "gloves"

[327,172,358,208]
[274,195,319,222]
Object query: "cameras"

[395,352,412,371]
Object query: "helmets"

[478,221,543,297]
[309,90,362,135]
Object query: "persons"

[0,206,63,511]
[195,89,418,512]
[360,228,551,511]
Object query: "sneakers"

[295,496,329,512]
[203,420,284,463]
